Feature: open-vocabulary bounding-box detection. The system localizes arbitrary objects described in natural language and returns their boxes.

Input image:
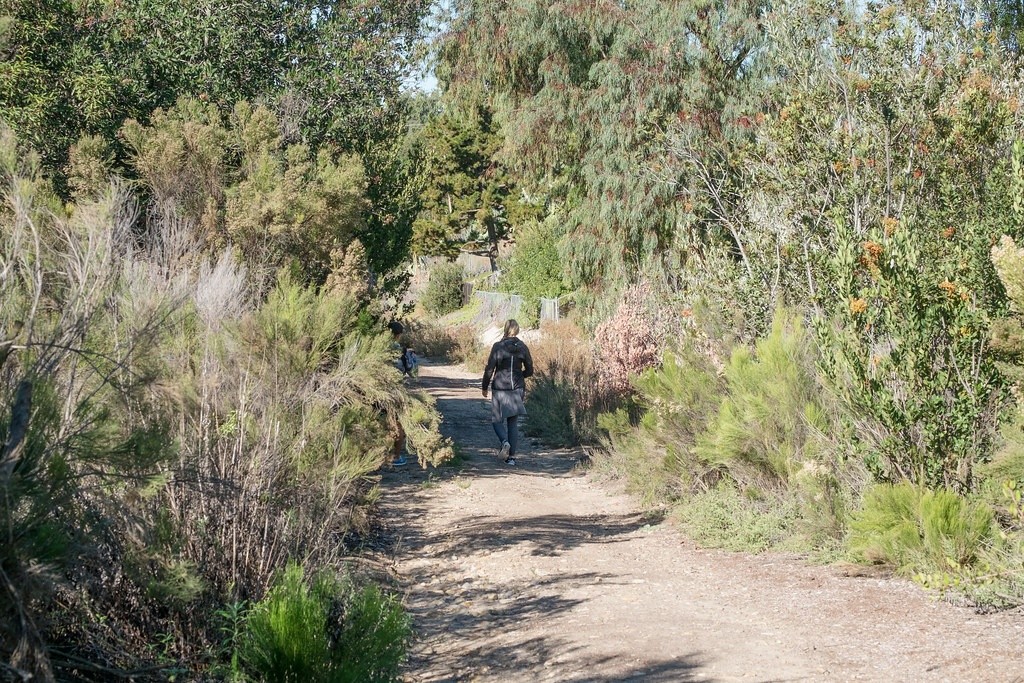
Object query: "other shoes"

[504,456,516,465]
[498,440,510,460]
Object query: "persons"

[481,319,534,466]
[387,322,418,465]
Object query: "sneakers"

[388,455,407,465]
